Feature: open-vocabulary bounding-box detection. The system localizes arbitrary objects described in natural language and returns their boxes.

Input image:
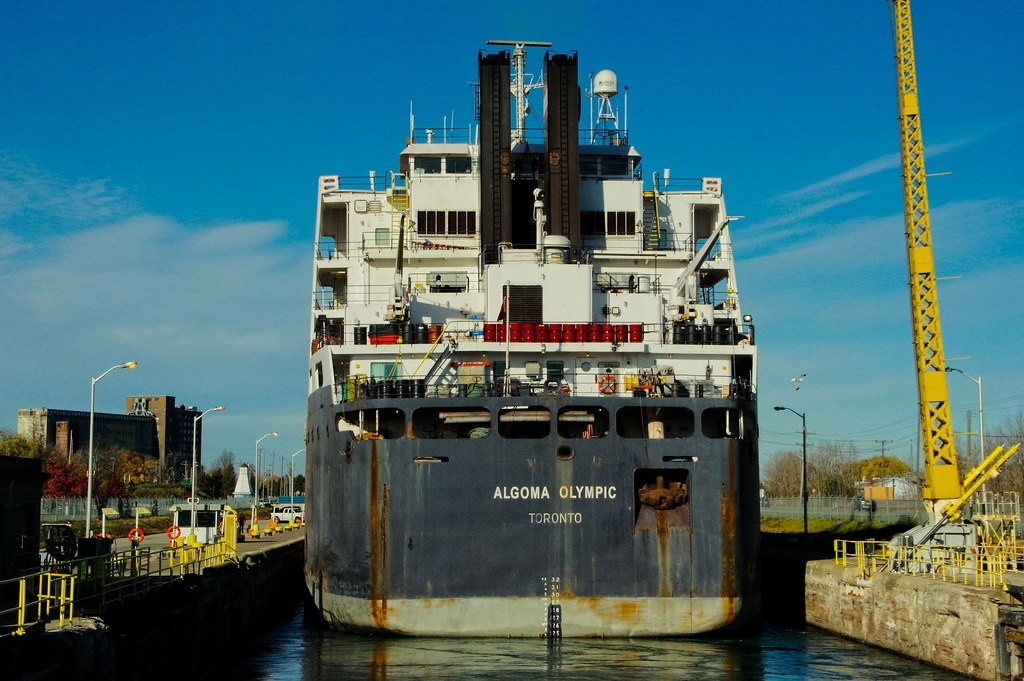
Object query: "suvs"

[853,496,876,511]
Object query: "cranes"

[889,1,977,545]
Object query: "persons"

[238,513,246,533]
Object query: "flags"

[497,296,506,321]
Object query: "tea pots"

[701,326,711,345]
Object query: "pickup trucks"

[270,508,304,523]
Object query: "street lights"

[291,449,306,521]
[946,367,990,543]
[254,432,279,522]
[193,405,225,536]
[774,406,809,535]
[86,360,140,540]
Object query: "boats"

[300,36,760,639]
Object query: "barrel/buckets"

[483,324,642,343]
[725,326,737,345]
[673,325,685,344]
[379,380,393,398]
[416,325,428,344]
[354,326,367,345]
[686,326,698,344]
[713,326,725,344]
[395,380,410,398]
[428,326,441,344]
[402,324,415,344]
[410,379,425,398]
[648,417,664,439]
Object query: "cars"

[761,498,769,507]
[258,499,270,507]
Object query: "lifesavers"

[599,376,617,395]
[167,526,181,539]
[97,533,113,544]
[128,529,144,543]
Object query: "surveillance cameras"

[533,188,545,199]
